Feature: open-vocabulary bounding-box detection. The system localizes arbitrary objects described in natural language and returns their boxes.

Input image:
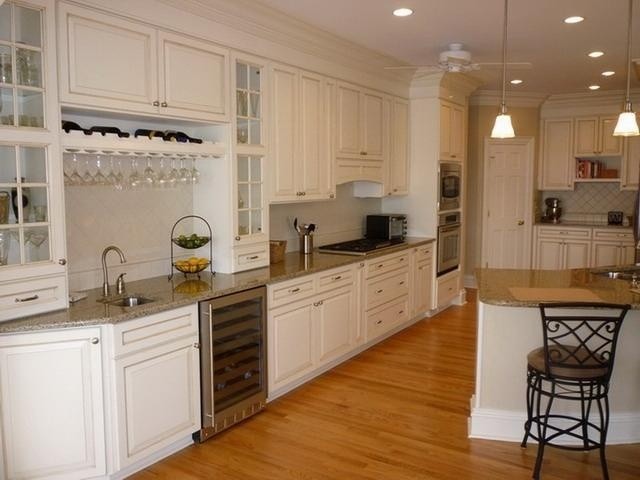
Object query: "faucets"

[100,244,126,297]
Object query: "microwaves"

[361,213,408,238]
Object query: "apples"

[174,234,209,248]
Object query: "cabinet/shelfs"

[532,224,593,270]
[532,114,640,191]
[593,227,636,268]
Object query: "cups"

[0,113,41,127]
[299,235,314,254]
[0,191,11,224]
[0,230,11,266]
[32,204,47,221]
[238,225,248,235]
[235,91,260,143]
[299,254,313,272]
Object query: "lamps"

[612,1,640,138]
[489,0,516,139]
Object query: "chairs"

[519,302,631,479]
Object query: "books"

[578,160,617,178]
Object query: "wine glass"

[27,232,50,262]
[12,230,32,256]
[63,146,202,192]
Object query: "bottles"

[0,38,41,89]
[238,191,244,209]
[12,177,32,224]
[61,118,204,144]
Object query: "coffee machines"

[541,196,562,224]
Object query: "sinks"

[596,270,639,281]
[96,291,161,309]
[621,267,640,275]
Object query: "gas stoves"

[317,237,409,257]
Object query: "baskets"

[172,260,211,274]
[171,235,211,250]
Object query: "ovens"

[436,212,462,279]
[436,163,462,211]
